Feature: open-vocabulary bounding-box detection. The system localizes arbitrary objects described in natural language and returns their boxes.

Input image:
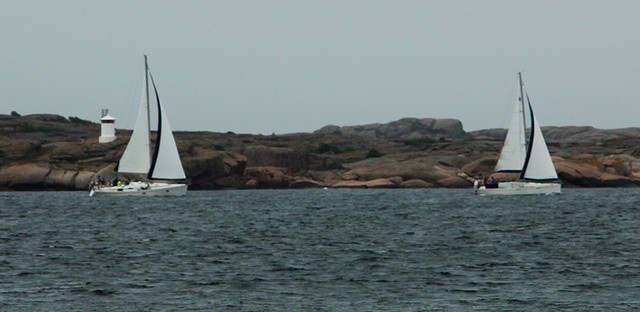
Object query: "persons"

[95,175,129,187]
[484,176,488,185]
[491,176,496,184]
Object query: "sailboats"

[471,71,561,198]
[90,53,189,198]
